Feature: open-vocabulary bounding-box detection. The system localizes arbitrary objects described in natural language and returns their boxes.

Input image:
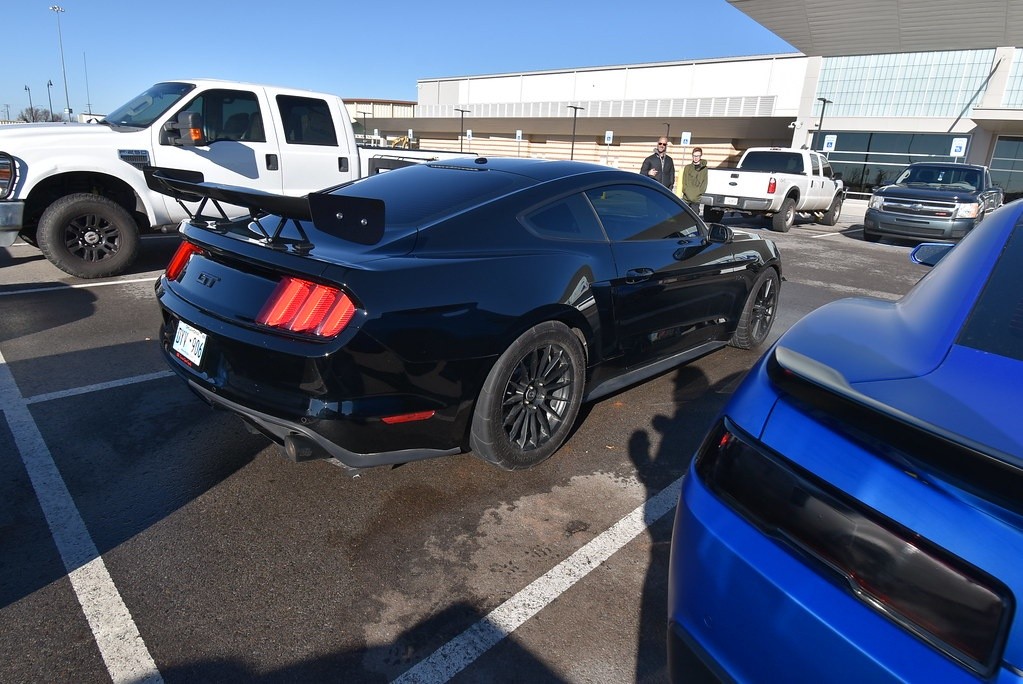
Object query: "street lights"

[565,104,585,161]
[23,85,36,123]
[47,79,56,122]
[357,111,373,146]
[455,108,472,154]
[814,97,833,154]
[50,5,73,123]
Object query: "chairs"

[919,171,934,182]
[244,112,263,139]
[965,172,978,188]
[287,112,309,141]
[218,112,249,140]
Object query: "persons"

[640,136,674,191]
[682,148,708,215]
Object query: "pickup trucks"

[0,79,479,280]
[700,147,845,232]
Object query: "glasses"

[658,143,667,146]
[693,155,701,157]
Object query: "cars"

[661,200,1023,684]
[138,157,783,474]
[863,162,1004,249]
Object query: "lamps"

[787,121,803,129]
